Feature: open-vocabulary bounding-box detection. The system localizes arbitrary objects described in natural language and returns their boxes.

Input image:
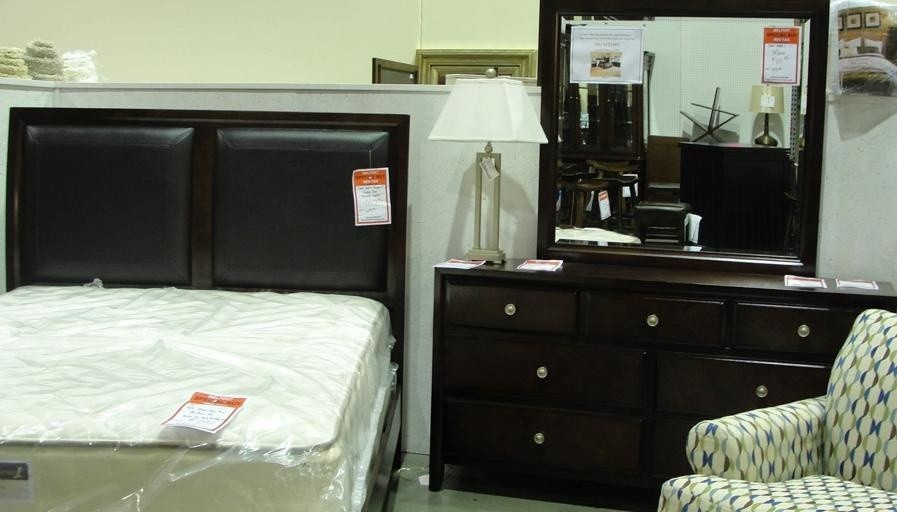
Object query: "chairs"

[557,156,640,233]
[634,134,690,243]
[658,308,897,510]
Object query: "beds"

[0,106,411,511]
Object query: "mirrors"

[536,0,831,285]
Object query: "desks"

[675,139,789,250]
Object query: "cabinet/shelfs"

[429,268,897,511]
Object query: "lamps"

[748,85,785,147]
[428,77,549,265]
